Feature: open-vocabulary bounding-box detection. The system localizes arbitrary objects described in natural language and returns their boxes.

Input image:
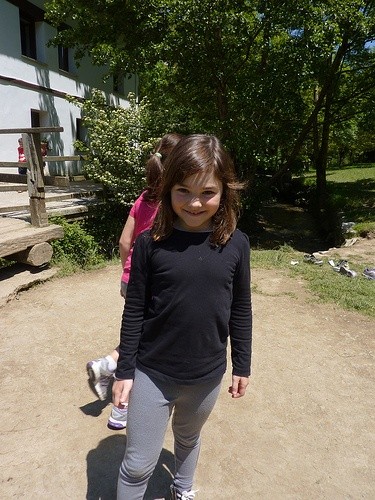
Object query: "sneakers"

[108,401,128,428]
[172,483,195,500]
[86,355,112,402]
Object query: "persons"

[87,133,182,430]
[116,134,253,500]
[17,137,27,175]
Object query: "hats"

[41,136,48,142]
